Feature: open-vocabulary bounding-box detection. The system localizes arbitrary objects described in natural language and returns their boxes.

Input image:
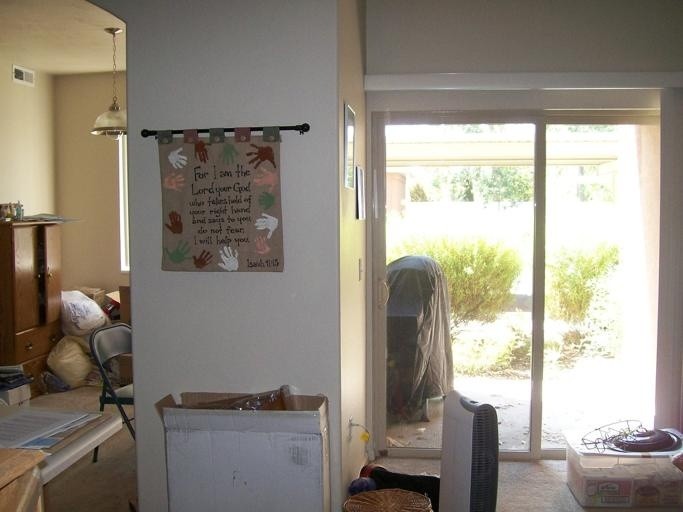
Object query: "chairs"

[89,321,137,466]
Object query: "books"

[1,408,101,448]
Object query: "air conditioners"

[437,389,501,511]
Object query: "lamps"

[92,27,126,139]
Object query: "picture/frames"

[341,100,356,191]
[354,166,368,223]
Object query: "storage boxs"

[154,386,332,512]
[0,383,33,408]
[563,440,683,512]
[116,352,132,387]
[76,283,130,327]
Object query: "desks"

[1,399,122,490]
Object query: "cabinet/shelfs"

[0,462,44,512]
[0,217,66,397]
